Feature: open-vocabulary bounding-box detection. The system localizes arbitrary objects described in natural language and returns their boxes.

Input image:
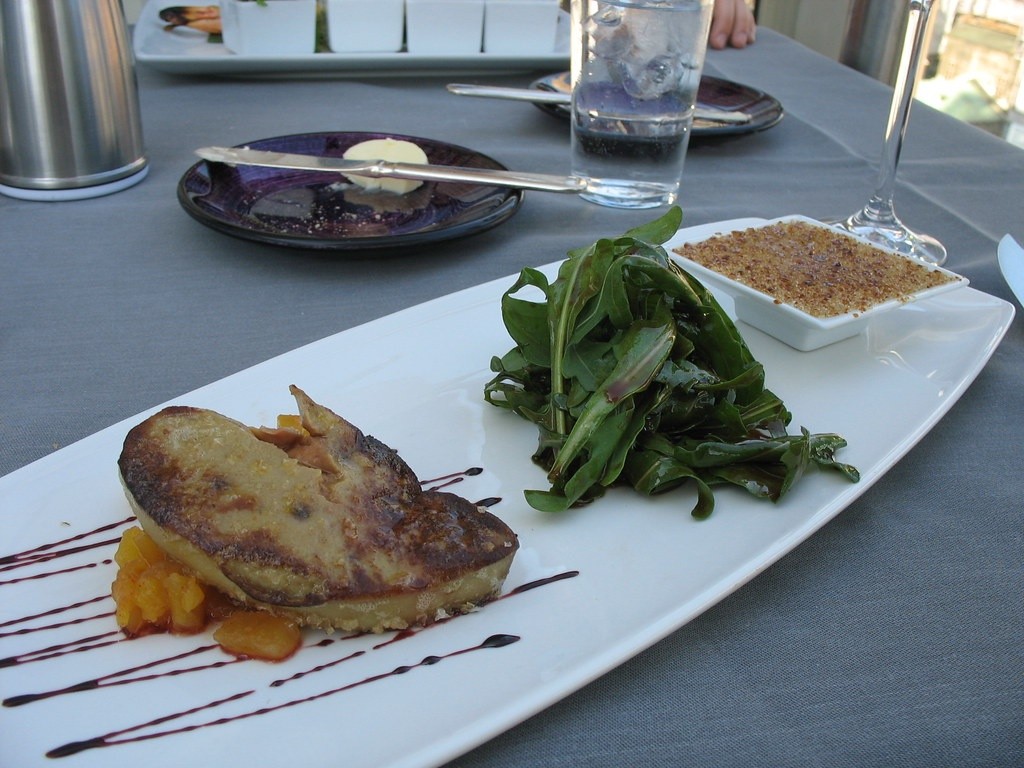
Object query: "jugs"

[1,0,149,201]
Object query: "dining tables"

[0,24,1023,768]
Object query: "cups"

[571,0,714,210]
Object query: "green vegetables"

[487,205,860,518]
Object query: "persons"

[709,0,756,50]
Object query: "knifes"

[194,146,584,195]
[447,82,752,123]
[997,233,1024,310]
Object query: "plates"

[178,131,525,247]
[0,215,1014,767]
[529,70,785,138]
[135,0,575,79]
[670,214,970,353]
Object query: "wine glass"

[819,0,947,267]
